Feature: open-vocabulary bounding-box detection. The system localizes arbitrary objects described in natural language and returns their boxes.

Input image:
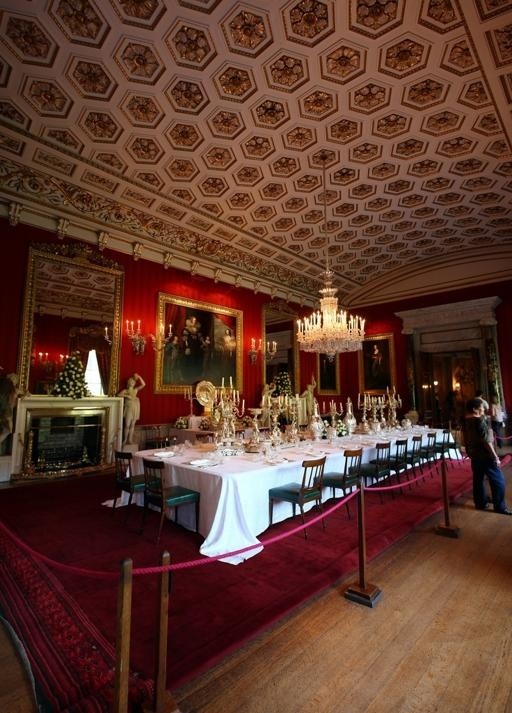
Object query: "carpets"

[0,454,511,711]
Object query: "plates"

[189,458,218,467]
[200,424,431,465]
[154,451,175,457]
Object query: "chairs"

[312,447,364,519]
[142,407,408,449]
[346,441,392,504]
[141,457,201,537]
[406,432,440,478]
[110,449,163,520]
[423,429,454,471]
[268,455,326,539]
[436,426,465,466]
[388,434,425,486]
[368,438,412,494]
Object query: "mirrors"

[14,244,126,397]
[262,299,299,395]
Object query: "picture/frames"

[357,333,396,396]
[154,293,245,395]
[317,353,340,395]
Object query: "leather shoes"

[476,504,489,509]
[500,508,512,514]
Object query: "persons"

[164,313,235,382]
[370,343,383,378]
[459,384,512,515]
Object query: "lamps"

[266,341,278,361]
[295,154,366,362]
[249,337,262,364]
[126,318,146,354]
[104,326,112,344]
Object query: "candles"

[215,377,403,412]
